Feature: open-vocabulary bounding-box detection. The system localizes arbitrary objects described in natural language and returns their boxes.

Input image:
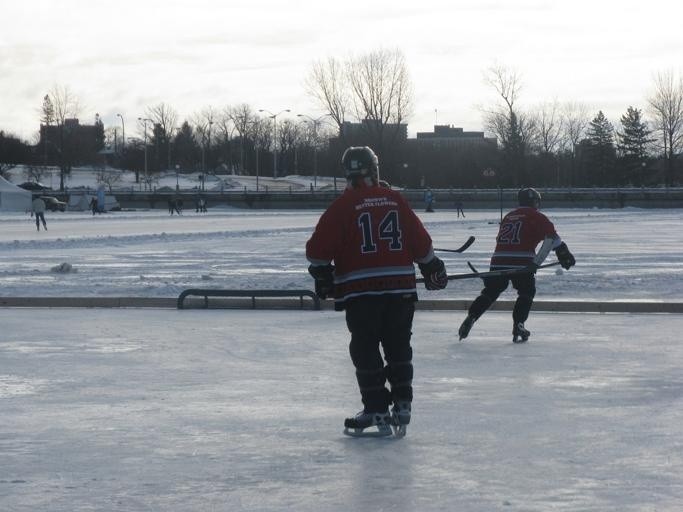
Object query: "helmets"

[517,187,542,212]
[340,145,378,188]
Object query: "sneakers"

[390,400,412,425]
[342,402,392,428]
[459,313,477,338]
[512,322,531,337]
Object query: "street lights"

[137,116,149,182]
[257,108,291,178]
[200,120,214,191]
[295,112,335,188]
[115,112,125,174]
[174,163,181,191]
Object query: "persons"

[456,185,575,342]
[306,147,448,437]
[166,193,208,216]
[454,193,465,218]
[88,196,101,215]
[423,187,434,212]
[30,195,50,232]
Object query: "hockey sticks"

[416,233,570,282]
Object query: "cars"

[13,181,53,190]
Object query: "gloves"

[418,257,447,291]
[307,263,341,301]
[553,241,576,270]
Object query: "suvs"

[32,196,68,212]
